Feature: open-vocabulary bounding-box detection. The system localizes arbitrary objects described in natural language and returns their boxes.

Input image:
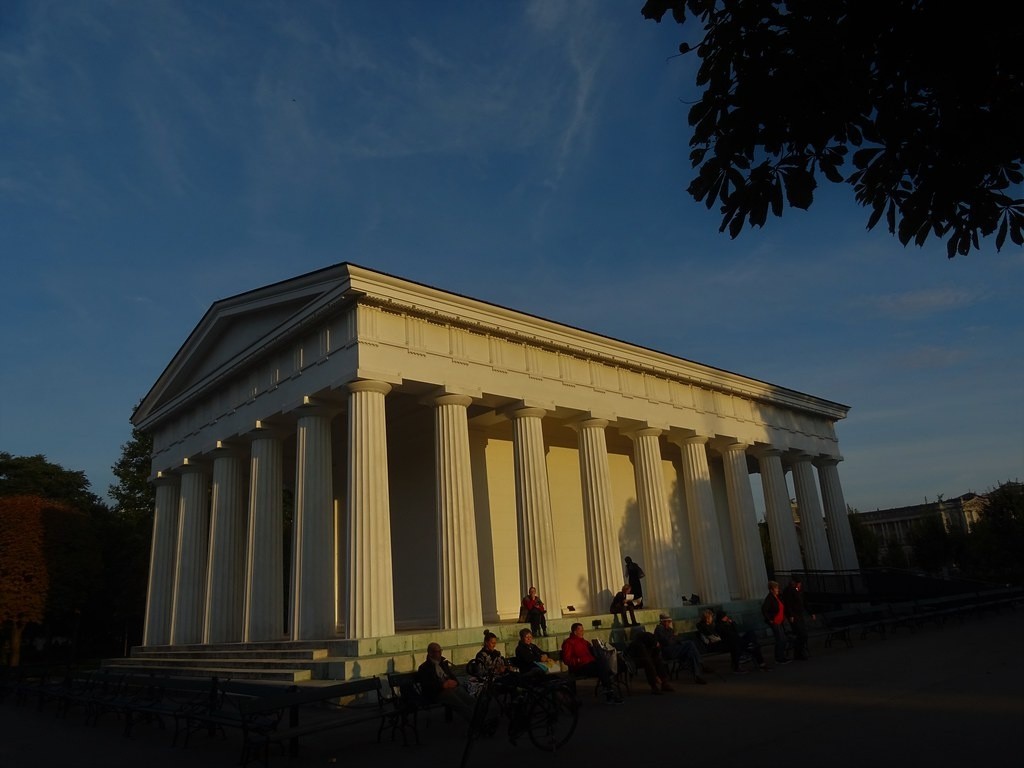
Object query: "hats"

[659,613,673,621]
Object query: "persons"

[417,623,625,728]
[624,556,645,608]
[519,587,549,637]
[610,575,816,694]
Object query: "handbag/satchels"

[636,566,645,580]
[533,651,568,674]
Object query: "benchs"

[0,586,1024,768]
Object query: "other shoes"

[694,676,707,684]
[733,667,745,672]
[543,633,549,637]
[651,687,664,696]
[702,669,714,677]
[794,655,808,661]
[632,621,640,626]
[538,633,542,637]
[624,623,631,627]
[759,664,773,671]
[776,658,793,664]
[600,695,625,705]
[662,685,675,691]
